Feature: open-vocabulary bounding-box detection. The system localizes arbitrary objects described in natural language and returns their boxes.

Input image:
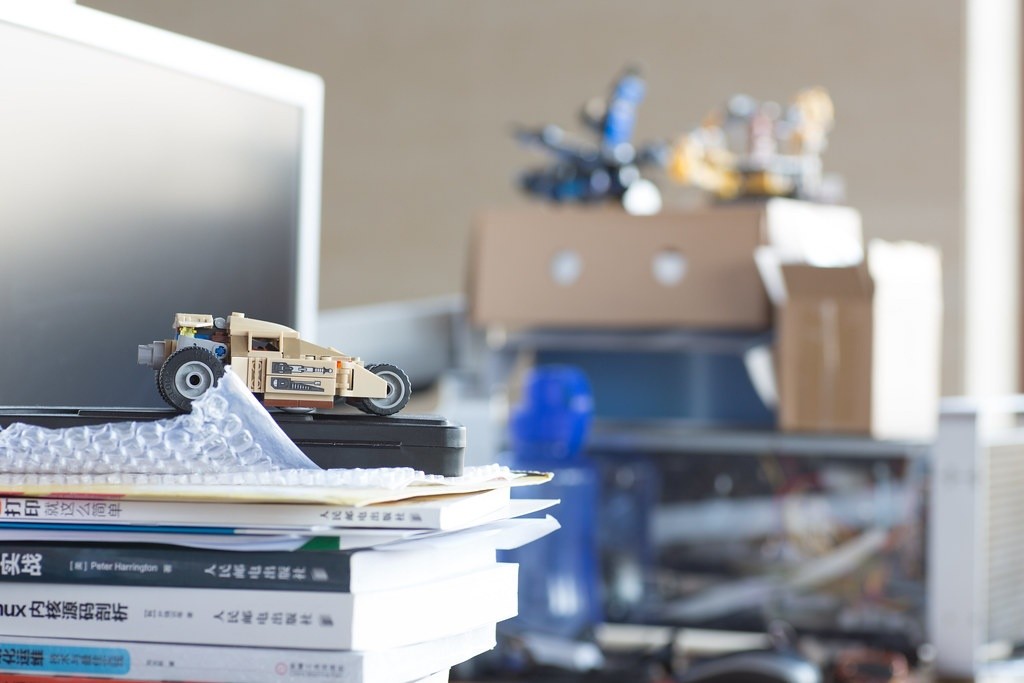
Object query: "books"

[0,465,561,683]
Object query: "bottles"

[497,359,604,641]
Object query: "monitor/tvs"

[0,0,326,418]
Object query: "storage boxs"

[462,197,775,335]
[758,191,942,452]
[0,407,469,476]
